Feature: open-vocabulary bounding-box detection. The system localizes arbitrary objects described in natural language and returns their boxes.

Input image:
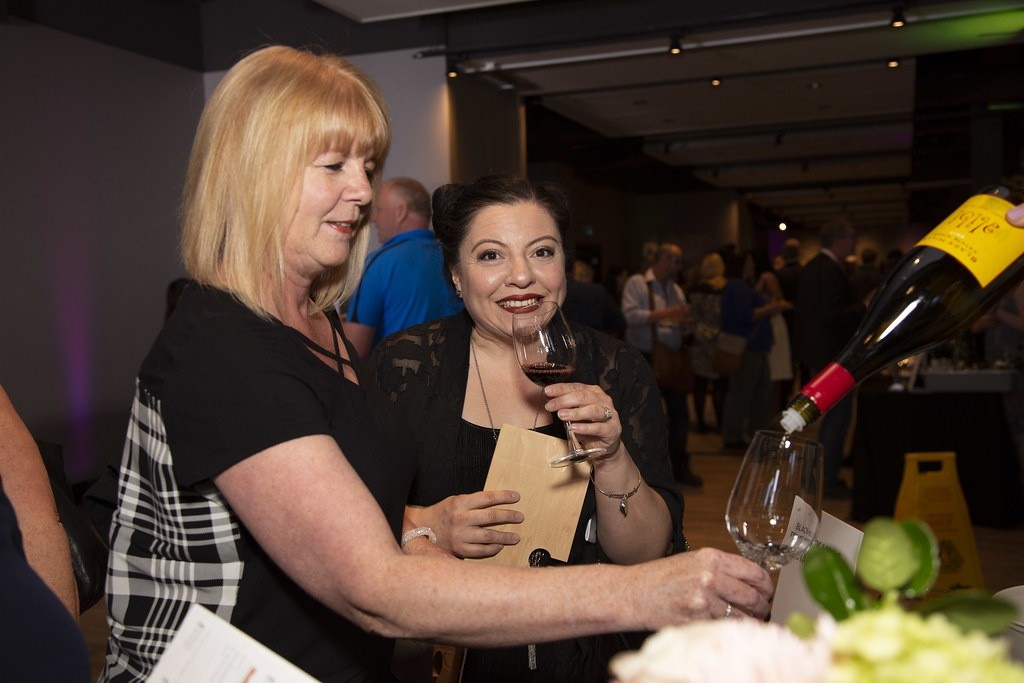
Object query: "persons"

[561,217,1023,492]
[0,384,82,683]
[367,170,686,683]
[339,177,467,358]
[102,44,773,683]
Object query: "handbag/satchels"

[714,332,748,375]
[648,349,678,396]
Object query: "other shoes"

[674,472,702,487]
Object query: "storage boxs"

[924,372,1013,393]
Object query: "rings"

[724,604,732,618]
[599,405,613,421]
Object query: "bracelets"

[401,527,437,547]
[590,464,642,518]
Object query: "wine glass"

[512,301,606,467]
[725,431,822,617]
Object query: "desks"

[854,393,1024,526]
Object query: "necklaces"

[469,334,548,452]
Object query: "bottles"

[781,184,1024,434]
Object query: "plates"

[993,585,1024,633]
